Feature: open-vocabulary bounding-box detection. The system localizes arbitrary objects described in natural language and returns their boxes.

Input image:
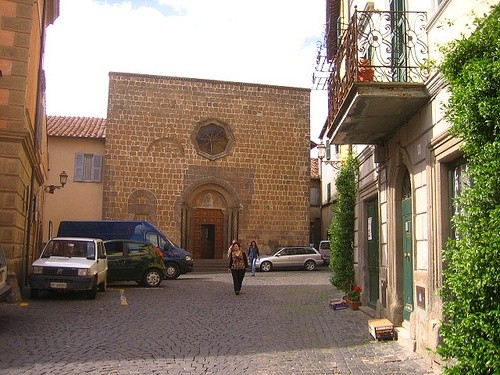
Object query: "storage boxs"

[368,319,393,339]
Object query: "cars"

[101,239,167,287]
[247,246,325,272]
[29,237,108,300]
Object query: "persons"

[227,242,250,295]
[247,240,260,276]
[228,240,246,258]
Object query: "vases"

[347,300,361,310]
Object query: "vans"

[318,240,332,264]
[56,220,195,279]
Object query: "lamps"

[44,171,68,194]
[317,141,341,169]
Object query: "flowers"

[346,286,362,301]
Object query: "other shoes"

[235,291,239,295]
[252,273,255,276]
